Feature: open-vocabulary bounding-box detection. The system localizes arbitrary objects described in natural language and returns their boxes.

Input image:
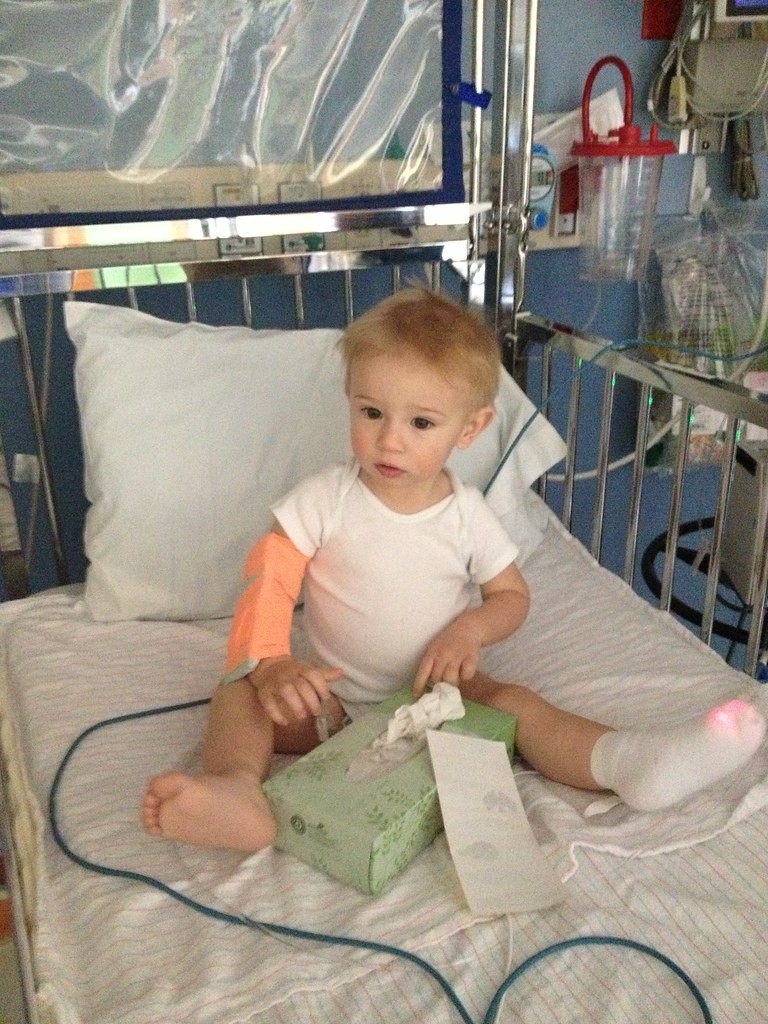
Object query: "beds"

[0,240,768,1024]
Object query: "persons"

[141,285,767,851]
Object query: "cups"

[570,125,676,281]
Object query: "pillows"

[62,299,572,625]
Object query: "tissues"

[262,675,518,897]
